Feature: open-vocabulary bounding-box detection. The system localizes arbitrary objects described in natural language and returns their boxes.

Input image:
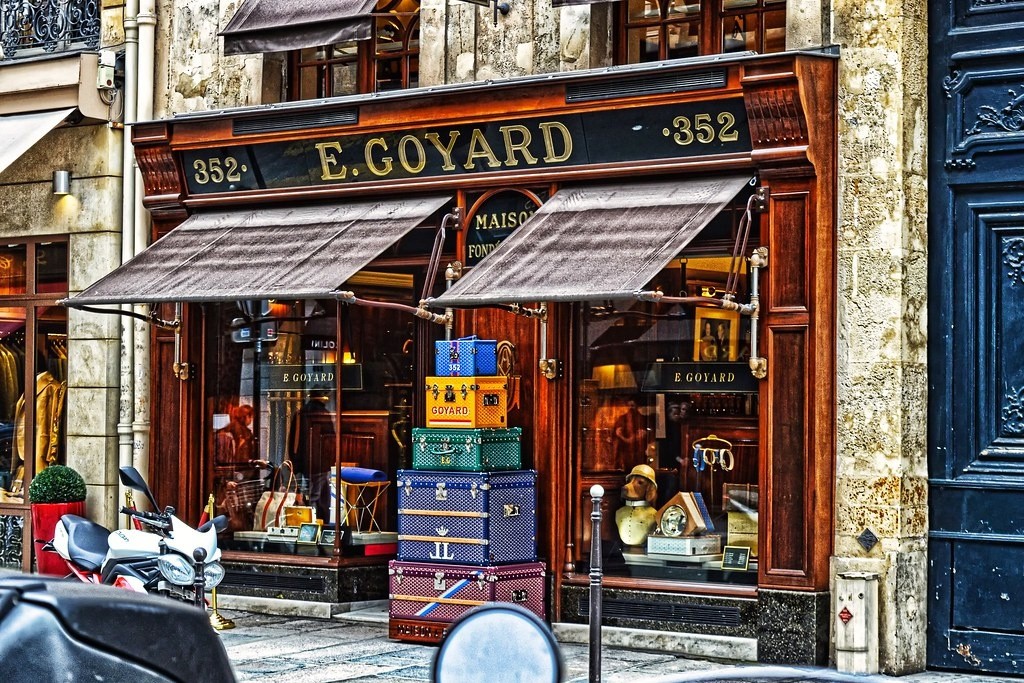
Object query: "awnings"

[57,196,454,307]
[427,177,753,315]
[0,108,75,176]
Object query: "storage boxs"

[268,505,317,537]
[434,335,498,376]
[412,427,522,471]
[425,376,508,429]
[396,469,538,566]
[722,483,759,512]
[388,560,547,644]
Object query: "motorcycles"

[34,466,230,626]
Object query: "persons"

[10,371,67,490]
[609,401,649,471]
[287,385,337,523]
[214,405,257,464]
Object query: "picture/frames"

[297,522,320,543]
[319,530,344,546]
[721,546,751,570]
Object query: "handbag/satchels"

[253,459,306,532]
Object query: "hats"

[626,464,658,490]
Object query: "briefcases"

[412,427,522,472]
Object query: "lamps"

[52,170,70,196]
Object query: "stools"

[328,478,392,534]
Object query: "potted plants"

[27,465,87,577]
